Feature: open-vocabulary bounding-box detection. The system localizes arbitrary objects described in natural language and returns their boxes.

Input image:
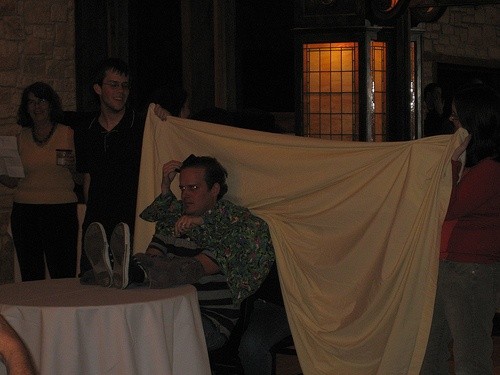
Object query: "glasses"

[449,115,458,121]
[27,98,47,105]
[179,182,205,192]
[102,81,132,90]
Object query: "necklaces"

[30,122,55,144]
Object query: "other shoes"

[106,221,131,289]
[84,221,111,288]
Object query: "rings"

[182,223,187,225]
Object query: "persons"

[0,313,37,375]
[420,86,500,375]
[83,153,290,375]
[0,60,189,284]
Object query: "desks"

[0,278,212,375]
